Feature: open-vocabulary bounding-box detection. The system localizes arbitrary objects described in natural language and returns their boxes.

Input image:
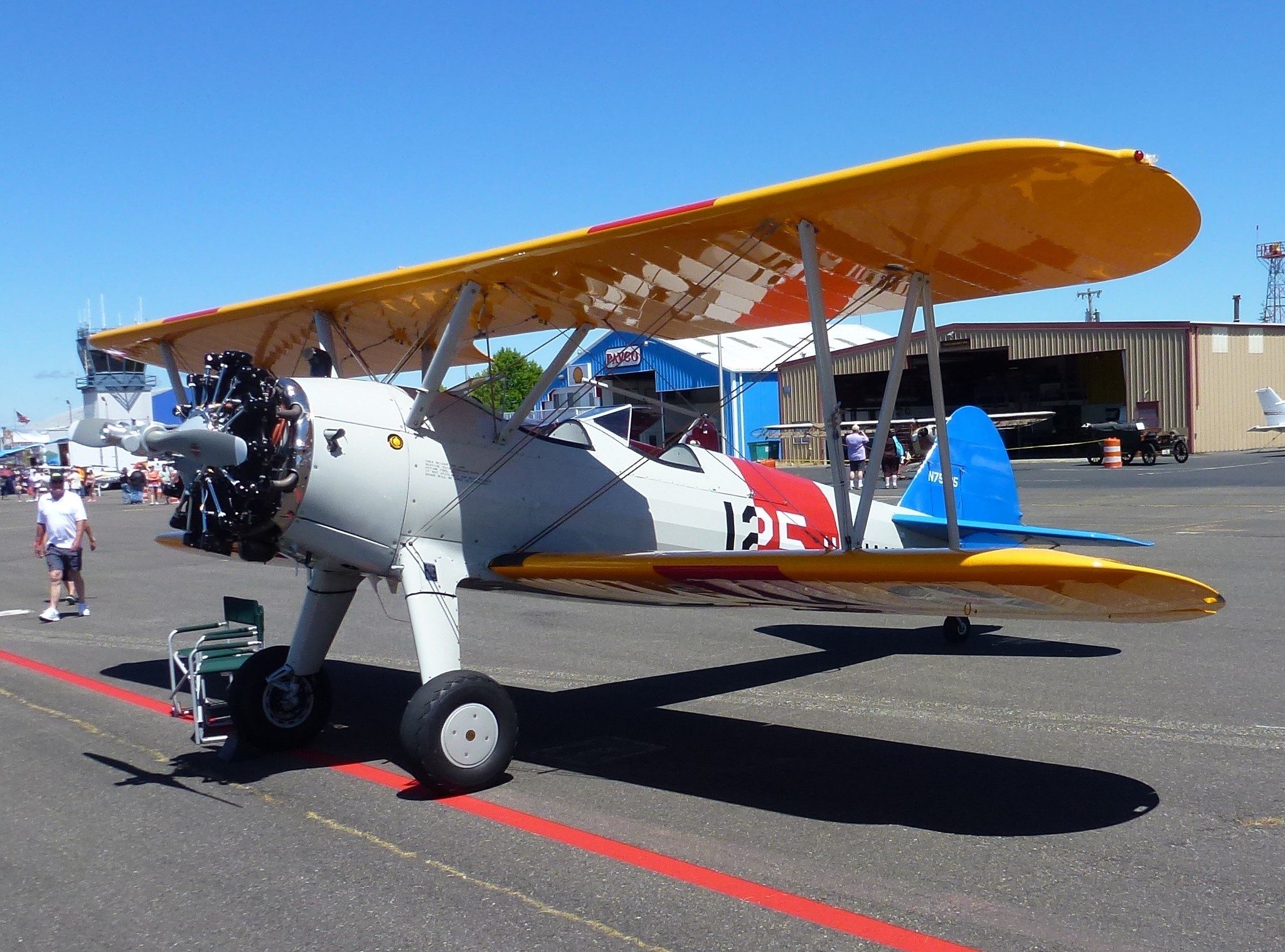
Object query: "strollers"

[127,471,145,505]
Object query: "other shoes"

[858,486,862,489]
[65,595,76,605]
[892,485,898,489]
[885,486,890,489]
[155,502,159,505]
[849,487,855,490]
[150,502,154,505]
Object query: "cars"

[1082,421,1189,466]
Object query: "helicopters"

[1246,387,1285,434]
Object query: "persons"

[846,425,869,489]
[881,430,900,488]
[2,464,171,622]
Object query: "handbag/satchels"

[893,436,904,457]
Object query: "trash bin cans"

[767,441,780,459]
[748,442,769,461]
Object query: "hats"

[148,465,154,468]
[51,472,63,481]
[852,424,860,430]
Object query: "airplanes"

[67,139,1227,795]
[762,411,1056,476]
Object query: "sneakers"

[39,608,60,622]
[78,602,90,616]
